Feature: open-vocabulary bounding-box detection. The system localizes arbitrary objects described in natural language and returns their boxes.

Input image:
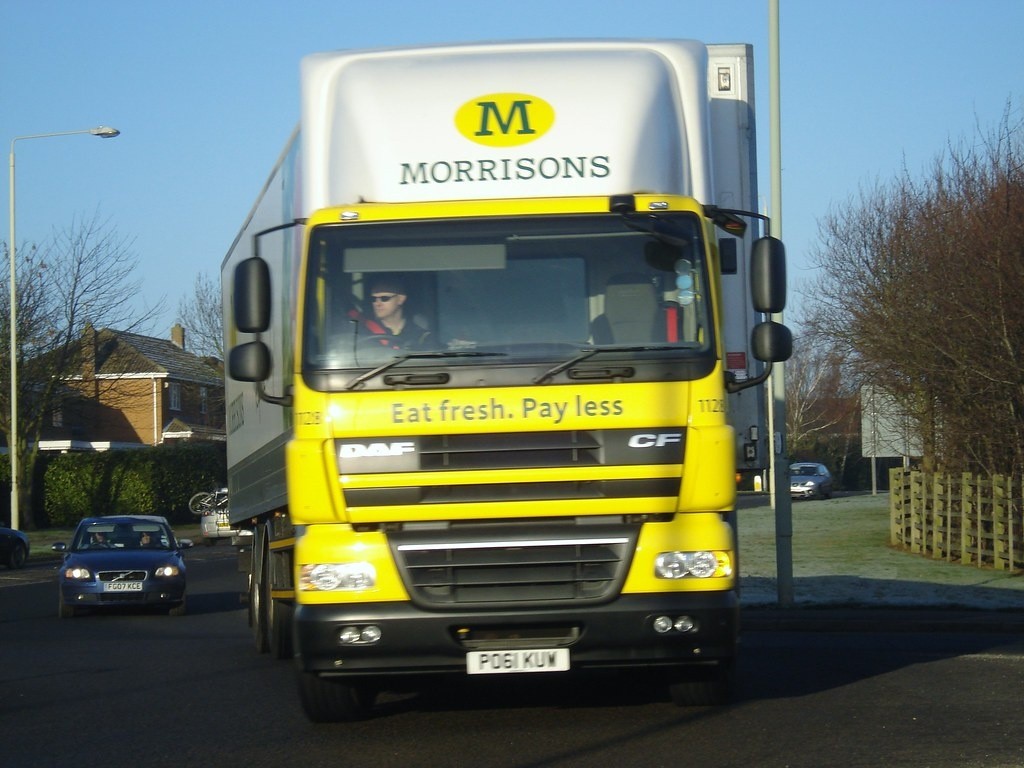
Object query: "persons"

[83,531,118,549]
[339,274,441,353]
[138,531,163,548]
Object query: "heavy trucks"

[215,42,789,727]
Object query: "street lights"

[9,126,123,534]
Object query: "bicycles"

[188,489,228,515]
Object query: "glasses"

[368,292,401,302]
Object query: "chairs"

[590,273,686,347]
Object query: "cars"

[0,527,31,570]
[789,462,833,501]
[51,512,197,616]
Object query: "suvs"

[201,488,259,548]
